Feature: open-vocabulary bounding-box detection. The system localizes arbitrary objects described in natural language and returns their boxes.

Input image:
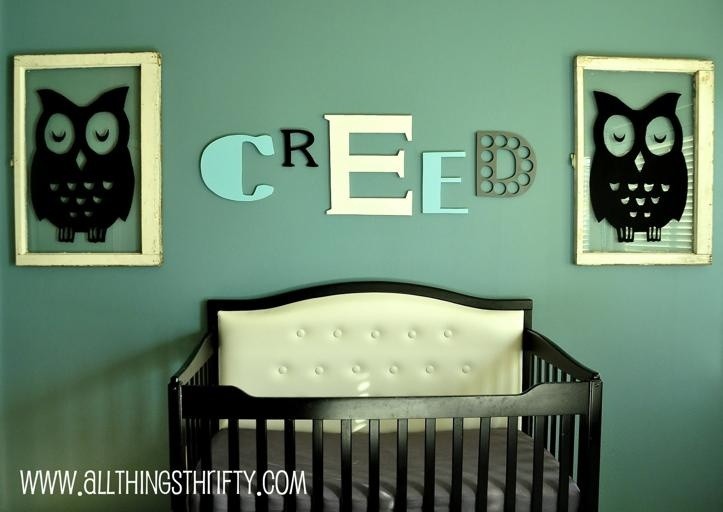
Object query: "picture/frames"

[569,50,716,264]
[12,49,165,267]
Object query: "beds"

[169,281,604,512]
